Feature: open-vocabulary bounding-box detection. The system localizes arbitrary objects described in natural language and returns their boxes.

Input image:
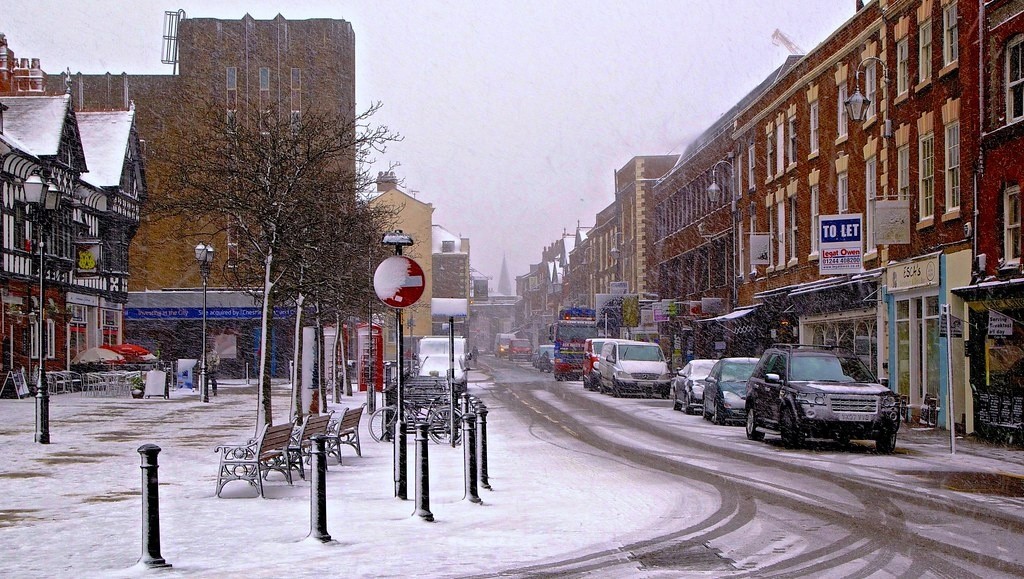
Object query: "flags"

[939,308,1015,339]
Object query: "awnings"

[694,308,755,324]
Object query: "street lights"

[24,170,63,446]
[195,241,215,404]
[382,229,416,500]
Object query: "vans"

[583,339,611,391]
[509,339,533,362]
[494,333,517,359]
[600,340,673,398]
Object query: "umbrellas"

[72,342,156,365]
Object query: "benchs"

[263,410,335,481]
[326,403,367,466]
[214,416,299,499]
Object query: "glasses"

[206,346,210,348]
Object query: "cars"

[533,345,556,374]
[419,335,473,389]
[673,359,718,414]
[701,357,761,426]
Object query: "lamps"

[609,231,625,261]
[708,161,733,202]
[845,56,890,122]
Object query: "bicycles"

[371,395,464,448]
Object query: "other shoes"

[214,392,217,396]
[204,399,209,402]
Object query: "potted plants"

[131,377,145,399]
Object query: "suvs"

[744,344,903,452]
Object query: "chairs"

[45,371,146,398]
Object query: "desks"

[97,374,122,397]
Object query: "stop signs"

[374,255,427,310]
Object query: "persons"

[199,343,220,396]
[471,346,479,365]
[405,348,414,366]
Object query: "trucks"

[549,316,598,380]
